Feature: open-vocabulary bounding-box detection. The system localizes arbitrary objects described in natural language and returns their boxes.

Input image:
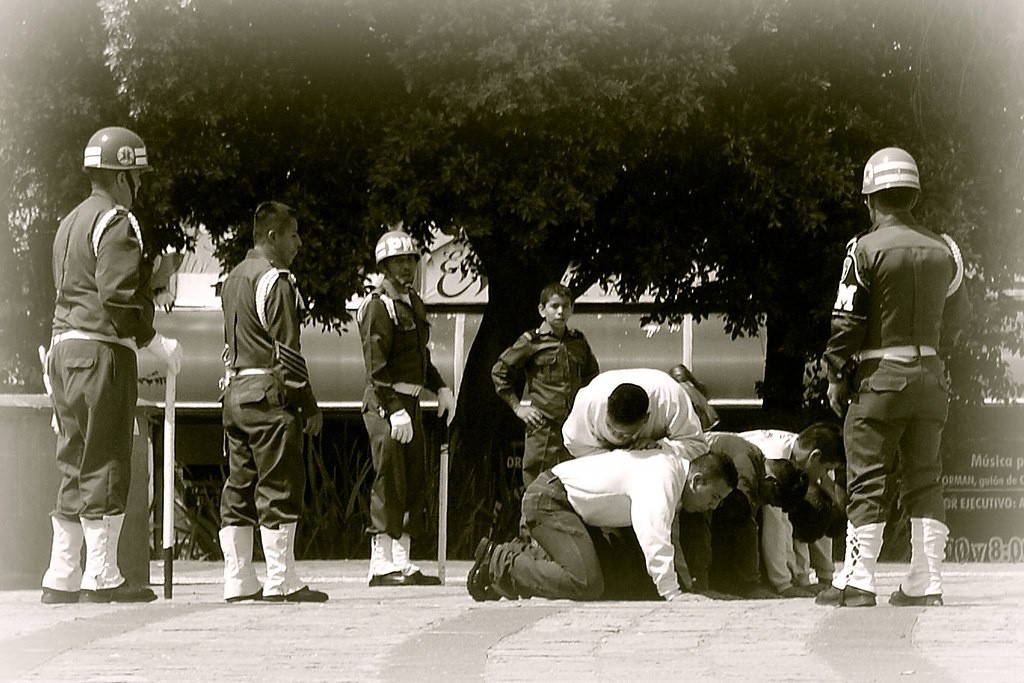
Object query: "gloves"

[390,409,413,443]
[437,387,456,426]
[145,333,183,376]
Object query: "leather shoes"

[41,588,81,603]
[227,588,264,603]
[78,579,158,604]
[263,586,328,603]
[406,570,441,585]
[889,591,943,606]
[467,537,501,602]
[369,571,416,586]
[815,585,877,608]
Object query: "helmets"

[861,147,920,194]
[84,126,149,170]
[375,230,422,263]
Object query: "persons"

[467,364,849,602]
[815,146,970,607]
[356,229,457,585]
[39,127,185,604]
[490,283,600,489]
[218,201,331,603]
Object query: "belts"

[851,345,936,361]
[232,368,273,376]
[393,382,422,397]
[53,329,139,361]
